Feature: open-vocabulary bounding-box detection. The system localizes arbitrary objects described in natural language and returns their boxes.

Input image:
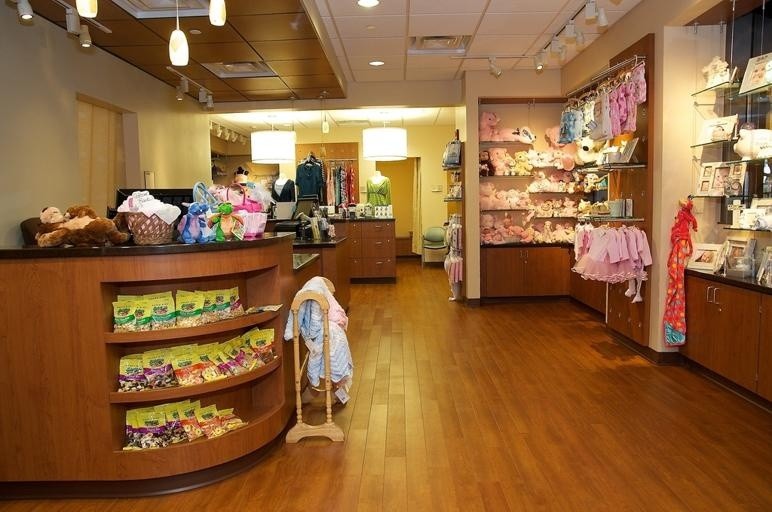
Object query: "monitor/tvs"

[115,188,193,231]
[302,194,318,199]
[291,198,319,221]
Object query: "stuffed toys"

[179,201,213,246]
[479,110,610,245]
[212,201,246,242]
[33,205,130,249]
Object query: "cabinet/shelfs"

[100,265,288,455]
[605,276,651,345]
[679,273,760,398]
[442,163,464,226]
[480,245,572,299]
[565,53,647,223]
[479,97,577,219]
[571,255,605,313]
[294,233,352,313]
[331,219,398,280]
[759,294,772,406]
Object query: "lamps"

[532,1,610,73]
[206,0,229,28]
[170,1,190,68]
[320,91,330,133]
[251,116,297,166]
[75,0,99,20]
[484,57,503,80]
[166,66,215,109]
[15,1,112,49]
[210,121,250,146]
[361,116,408,162]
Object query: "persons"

[272,175,296,203]
[366,170,391,215]
[716,168,729,187]
[752,62,766,82]
[697,250,715,263]
[234,166,249,186]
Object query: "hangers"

[568,56,644,107]
[577,213,642,230]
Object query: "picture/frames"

[619,136,641,164]
[686,50,771,289]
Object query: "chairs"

[421,226,449,265]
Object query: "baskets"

[124,209,174,245]
[193,182,218,240]
[227,183,267,236]
